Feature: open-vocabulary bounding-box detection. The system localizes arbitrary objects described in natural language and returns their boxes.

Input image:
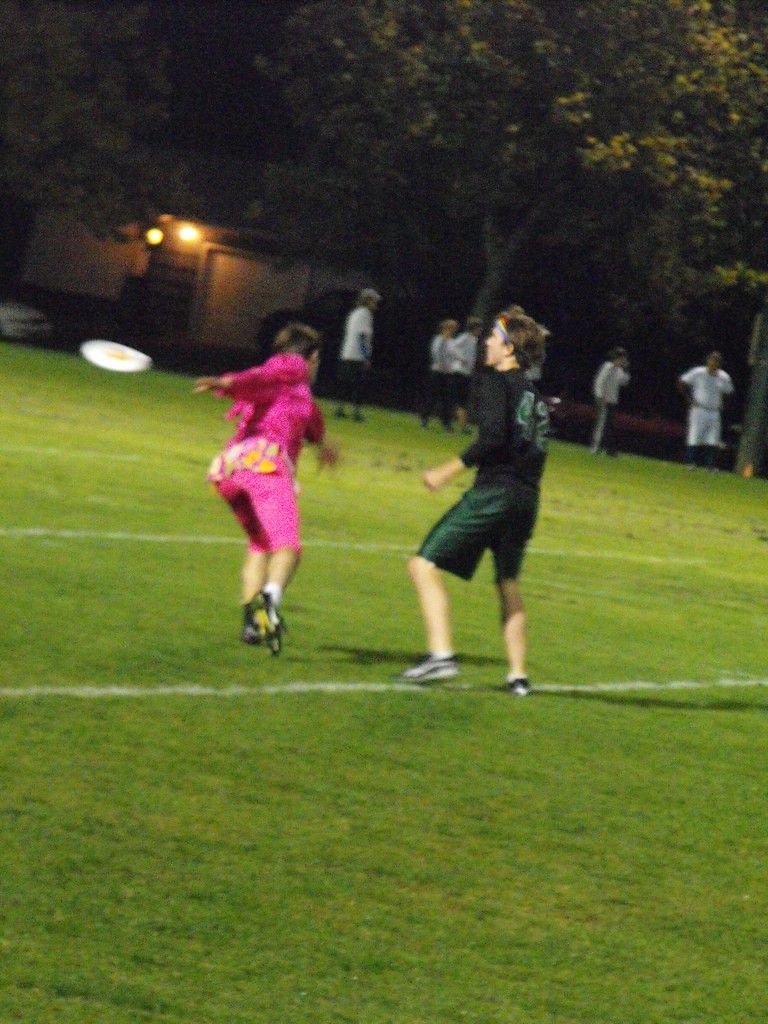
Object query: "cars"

[537,362,743,470]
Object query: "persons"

[193,325,338,658]
[334,288,380,422]
[677,351,734,469]
[403,306,549,699]
[590,347,629,457]
[448,316,484,433]
[420,318,458,427]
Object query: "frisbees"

[81,338,154,373]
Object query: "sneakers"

[243,623,261,644]
[252,591,281,653]
[401,657,459,682]
[490,679,529,697]
